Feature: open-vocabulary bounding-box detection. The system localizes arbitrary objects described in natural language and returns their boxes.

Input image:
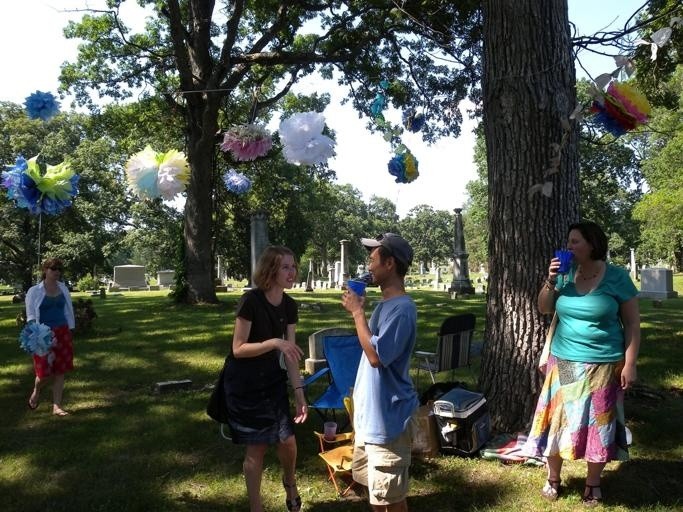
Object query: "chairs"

[411,312,478,393]
[313,395,369,500]
[294,330,375,434]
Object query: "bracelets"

[543,277,559,293]
[292,386,304,392]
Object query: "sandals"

[584,483,605,504]
[541,478,563,503]
[281,473,303,512]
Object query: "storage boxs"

[432,385,492,458]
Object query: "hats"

[358,231,416,271]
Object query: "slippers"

[27,397,41,411]
[51,409,70,417]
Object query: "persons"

[515,221,641,505]
[341,232,421,512]
[24,257,76,417]
[223,244,308,512]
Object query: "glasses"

[46,266,62,272]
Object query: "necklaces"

[577,262,604,281]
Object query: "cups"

[324,422,337,441]
[348,280,368,296]
[555,249,574,272]
